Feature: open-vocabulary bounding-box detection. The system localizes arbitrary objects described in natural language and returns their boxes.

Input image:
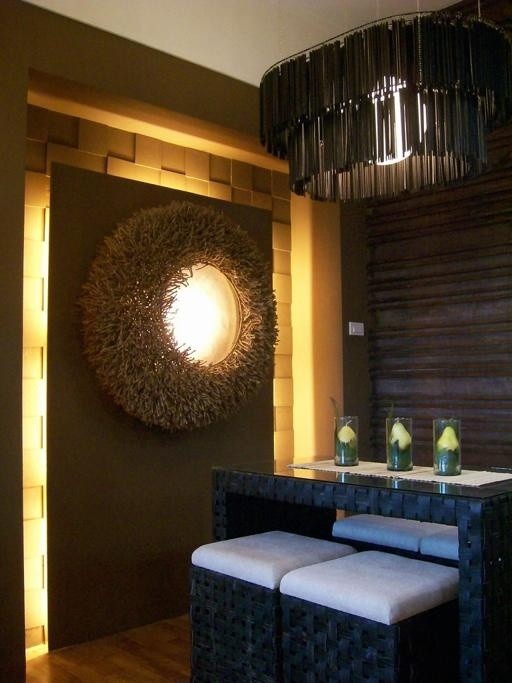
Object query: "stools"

[282,551,460,682]
[189,532,355,683]
[420,526,460,567]
[331,513,452,560]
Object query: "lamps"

[258,0,511,200]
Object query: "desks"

[210,460,511,683]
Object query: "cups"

[431,417,463,474]
[333,414,361,465]
[384,416,414,471]
